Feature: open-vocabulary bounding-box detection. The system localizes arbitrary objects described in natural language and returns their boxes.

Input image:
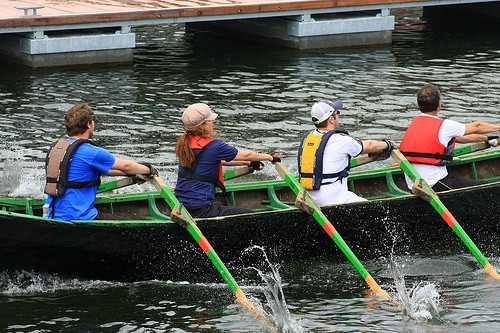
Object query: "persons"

[298,98,400,207]
[400,84,500,195]
[42,102,160,221]
[173,103,283,219]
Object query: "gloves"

[143,164,159,179]
[486,136,500,147]
[382,138,398,153]
[129,173,146,185]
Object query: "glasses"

[333,111,340,116]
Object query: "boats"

[0,150,500,283]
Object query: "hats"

[311,99,343,125]
[180,103,219,129]
